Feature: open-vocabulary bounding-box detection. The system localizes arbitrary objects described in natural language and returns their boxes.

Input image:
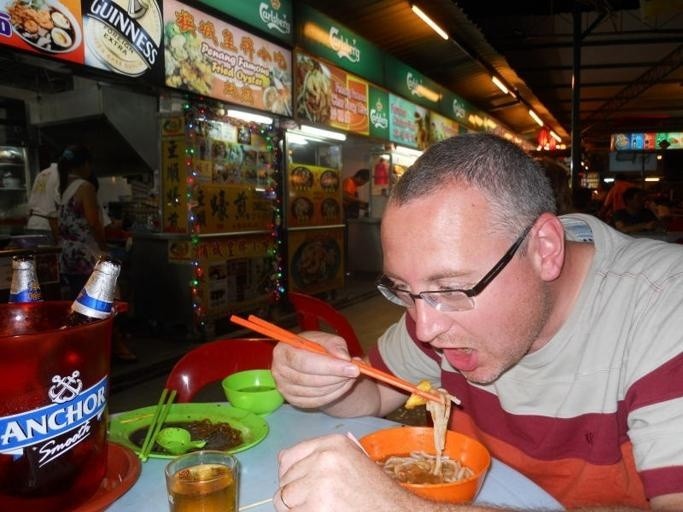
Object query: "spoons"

[154,426,206,455]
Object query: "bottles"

[3,256,123,497]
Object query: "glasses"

[373,216,540,315]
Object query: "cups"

[162,449,241,512]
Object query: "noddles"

[377,388,477,485]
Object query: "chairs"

[288,290,365,356]
[164,335,278,403]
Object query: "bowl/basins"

[220,368,285,416]
[352,425,491,505]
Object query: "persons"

[530,158,577,216]
[48,142,138,366]
[24,157,61,231]
[591,165,678,240]
[271,132,683,512]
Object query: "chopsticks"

[136,386,177,462]
[229,313,451,405]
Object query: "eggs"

[51,11,71,48]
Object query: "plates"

[320,198,340,218]
[290,167,314,193]
[290,236,341,289]
[319,170,339,193]
[290,196,314,220]
[72,440,142,512]
[7,0,82,55]
[106,402,269,459]
